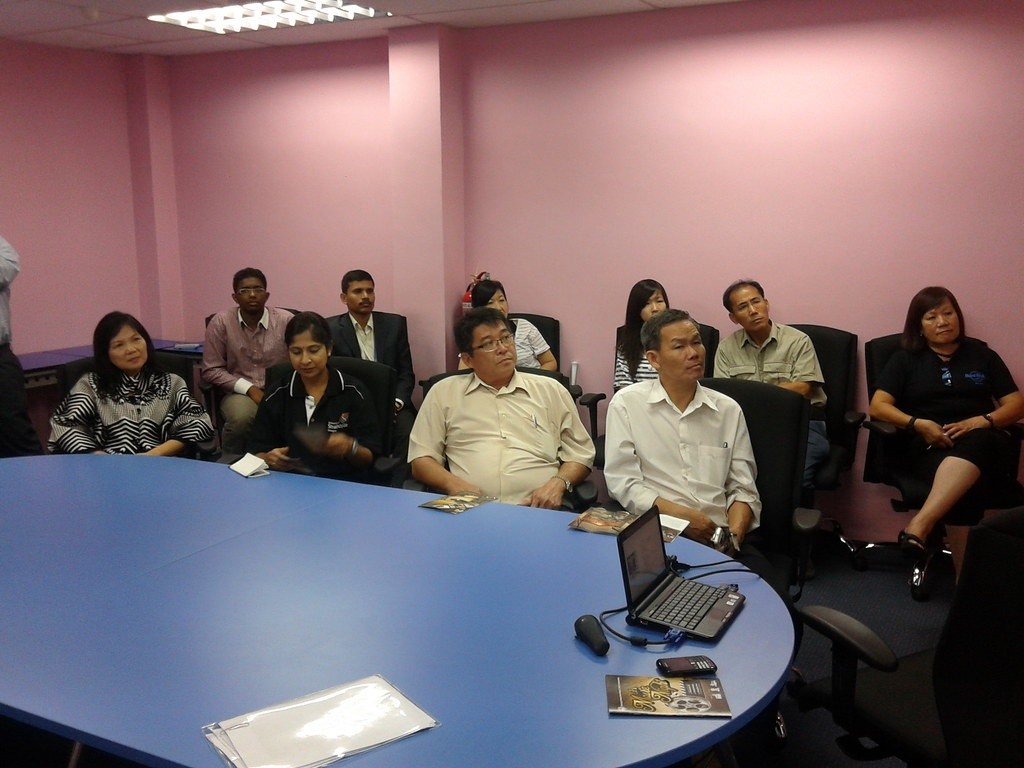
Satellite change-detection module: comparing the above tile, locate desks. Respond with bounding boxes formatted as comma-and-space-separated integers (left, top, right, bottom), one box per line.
16, 337, 204, 390
0, 454, 797, 768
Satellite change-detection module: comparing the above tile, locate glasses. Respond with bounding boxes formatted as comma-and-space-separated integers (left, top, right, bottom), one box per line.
472, 333, 514, 352
236, 288, 265, 295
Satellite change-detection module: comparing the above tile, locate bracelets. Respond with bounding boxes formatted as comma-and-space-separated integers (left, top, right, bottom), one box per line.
906, 416, 917, 434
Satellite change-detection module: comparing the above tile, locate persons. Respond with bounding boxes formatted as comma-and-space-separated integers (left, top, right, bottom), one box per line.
604, 309, 804, 663
713, 280, 830, 579
47, 311, 215, 457
612, 279, 670, 390
202, 268, 296, 454
867, 286, 1024, 585
246, 312, 385, 488
0, 237, 44, 458
324, 269, 418, 489
458, 280, 557, 371
407, 306, 595, 509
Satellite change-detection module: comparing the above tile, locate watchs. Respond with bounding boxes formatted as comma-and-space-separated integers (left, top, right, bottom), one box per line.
982, 413, 994, 428
347, 439, 358, 459
551, 474, 574, 495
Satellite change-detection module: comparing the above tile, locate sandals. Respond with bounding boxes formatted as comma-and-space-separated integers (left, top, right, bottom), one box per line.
897, 529, 926, 556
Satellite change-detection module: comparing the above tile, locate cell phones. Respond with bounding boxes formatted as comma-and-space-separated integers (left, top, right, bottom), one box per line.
656, 655, 717, 677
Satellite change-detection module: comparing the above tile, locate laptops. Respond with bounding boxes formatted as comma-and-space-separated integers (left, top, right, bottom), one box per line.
616, 505, 747, 643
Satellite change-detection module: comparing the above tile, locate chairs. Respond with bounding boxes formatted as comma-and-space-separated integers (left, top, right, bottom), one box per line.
195, 308, 1024, 768
